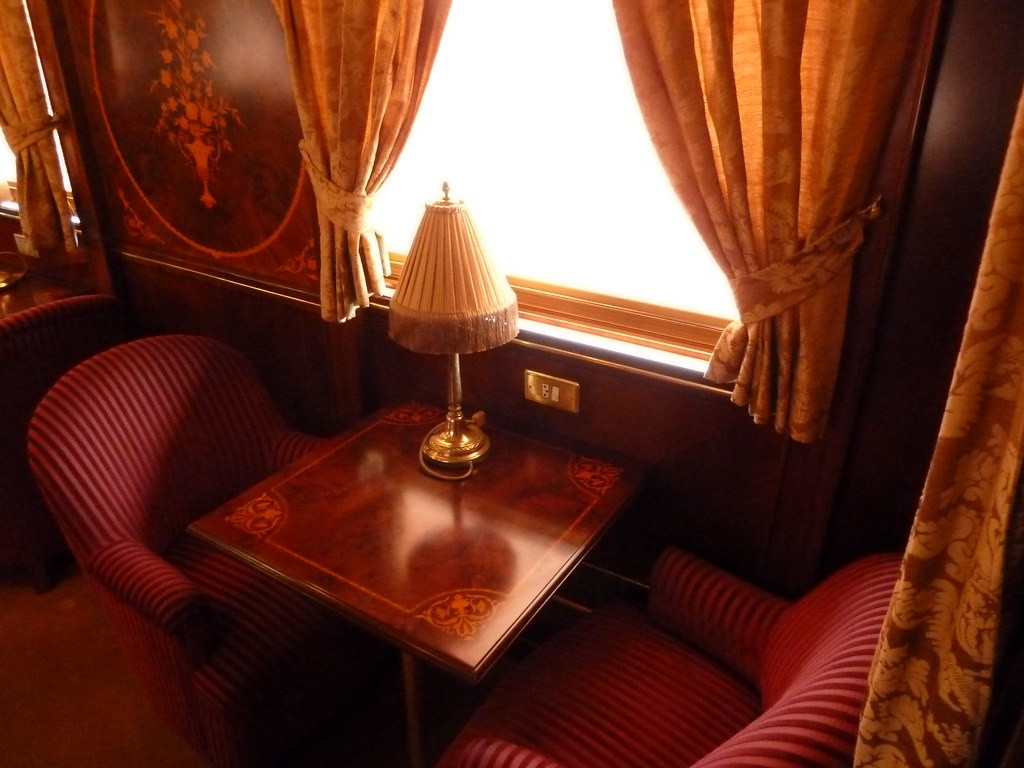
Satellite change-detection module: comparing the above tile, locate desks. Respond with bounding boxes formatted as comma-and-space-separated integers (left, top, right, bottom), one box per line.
184, 395, 654, 768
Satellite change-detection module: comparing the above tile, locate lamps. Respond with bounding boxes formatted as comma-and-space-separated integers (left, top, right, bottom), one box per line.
384, 181, 518, 479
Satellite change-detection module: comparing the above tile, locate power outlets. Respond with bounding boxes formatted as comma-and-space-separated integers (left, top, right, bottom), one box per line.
524, 369, 580, 415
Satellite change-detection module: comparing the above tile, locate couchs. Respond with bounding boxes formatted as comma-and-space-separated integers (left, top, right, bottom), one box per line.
27, 337, 355, 768
0, 293, 126, 593
439, 548, 907, 768
